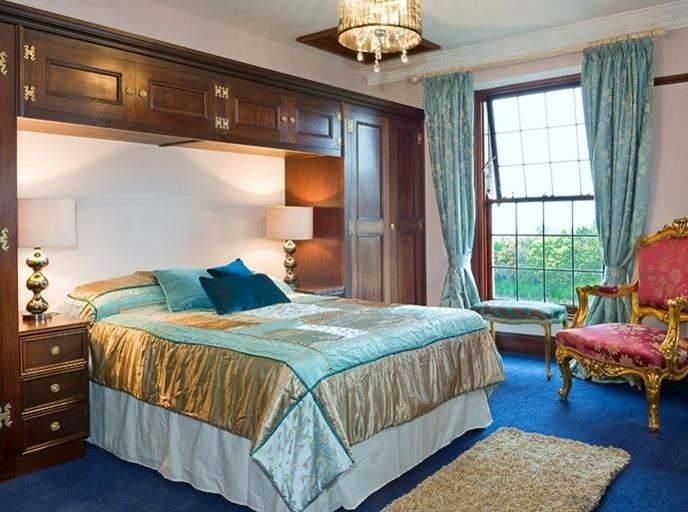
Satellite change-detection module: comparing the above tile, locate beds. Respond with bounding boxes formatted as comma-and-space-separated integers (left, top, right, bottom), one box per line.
55, 269, 506, 512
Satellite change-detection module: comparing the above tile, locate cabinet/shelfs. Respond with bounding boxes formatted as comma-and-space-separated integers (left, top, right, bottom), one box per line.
283, 103, 424, 304
159, 79, 339, 162
18, 28, 221, 149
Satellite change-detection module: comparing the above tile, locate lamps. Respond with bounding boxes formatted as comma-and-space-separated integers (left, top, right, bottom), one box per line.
336, 0, 422, 73
264, 205, 315, 289
16, 198, 75, 322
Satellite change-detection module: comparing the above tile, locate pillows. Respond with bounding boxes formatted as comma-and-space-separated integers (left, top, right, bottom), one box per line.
154, 266, 212, 313
208, 259, 253, 278
199, 272, 289, 314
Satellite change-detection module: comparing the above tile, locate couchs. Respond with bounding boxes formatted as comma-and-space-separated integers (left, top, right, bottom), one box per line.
554, 216, 688, 435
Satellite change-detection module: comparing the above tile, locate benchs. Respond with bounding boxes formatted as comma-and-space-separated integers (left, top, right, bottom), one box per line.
466, 301, 567, 379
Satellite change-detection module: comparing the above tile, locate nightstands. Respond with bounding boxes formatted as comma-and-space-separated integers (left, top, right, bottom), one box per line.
19, 312, 91, 477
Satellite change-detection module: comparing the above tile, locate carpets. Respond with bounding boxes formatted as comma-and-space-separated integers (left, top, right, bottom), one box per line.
375, 423, 631, 512
